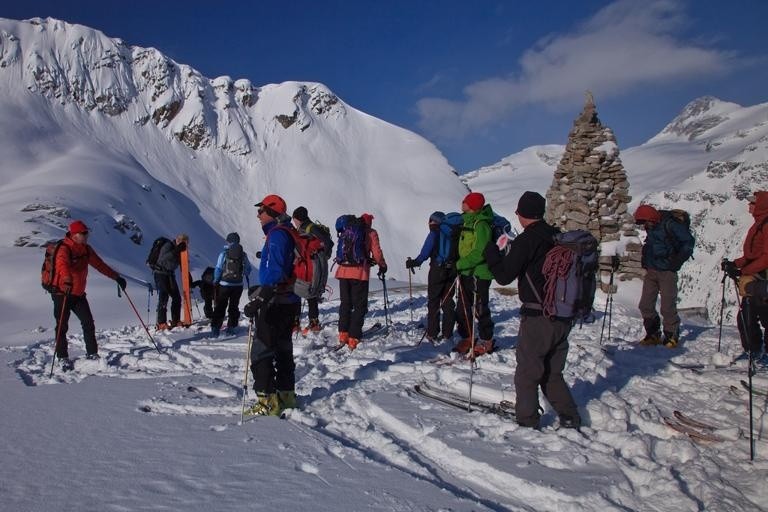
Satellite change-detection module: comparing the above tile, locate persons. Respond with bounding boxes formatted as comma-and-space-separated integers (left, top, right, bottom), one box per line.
290, 206, 331, 332
152, 233, 191, 329
406, 193, 496, 359
632, 202, 696, 351
480, 191, 582, 429
199, 233, 252, 335
239, 195, 305, 419
736, 273, 768, 302
730, 188, 768, 370
48, 219, 128, 373
335, 212, 388, 351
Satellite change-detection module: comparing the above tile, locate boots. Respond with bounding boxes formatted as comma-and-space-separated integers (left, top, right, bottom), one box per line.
277, 390, 297, 409
245, 391, 280, 415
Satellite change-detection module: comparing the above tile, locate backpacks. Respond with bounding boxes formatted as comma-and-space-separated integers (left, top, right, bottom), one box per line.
331, 215, 370, 272
221, 242, 244, 283
473, 215, 518, 257
662, 209, 695, 261
436, 212, 465, 265
305, 220, 334, 260
146, 237, 175, 274
266, 225, 328, 299
526, 228, 599, 323
42, 239, 73, 291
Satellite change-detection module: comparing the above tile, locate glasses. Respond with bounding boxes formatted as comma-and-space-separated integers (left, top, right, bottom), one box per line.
258, 209, 266, 215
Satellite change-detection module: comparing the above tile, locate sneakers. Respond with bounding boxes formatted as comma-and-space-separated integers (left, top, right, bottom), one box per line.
639, 329, 662, 345
158, 317, 239, 339
86, 353, 100, 359
292, 317, 358, 349
756, 354, 768, 367
737, 351, 761, 360
58, 357, 73, 370
426, 330, 494, 356
663, 333, 678, 347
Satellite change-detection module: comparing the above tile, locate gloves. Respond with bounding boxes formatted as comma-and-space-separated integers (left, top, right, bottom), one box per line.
448, 261, 460, 273
368, 256, 376, 266
721, 261, 736, 272
379, 265, 387, 273
172, 241, 186, 257
116, 276, 126, 290
64, 281, 73, 295
244, 284, 275, 316
406, 260, 416, 268
213, 280, 219, 286
725, 266, 742, 276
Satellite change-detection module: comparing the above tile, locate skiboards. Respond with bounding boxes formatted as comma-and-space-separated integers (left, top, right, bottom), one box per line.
729, 379, 767, 400
181, 249, 192, 326
665, 411, 730, 444
667, 359, 750, 376
414, 382, 563, 431
332, 323, 391, 353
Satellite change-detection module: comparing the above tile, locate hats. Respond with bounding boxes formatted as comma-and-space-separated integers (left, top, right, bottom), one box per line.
70, 221, 90, 234
745, 195, 757, 203
254, 195, 286, 215
517, 191, 545, 219
227, 232, 240, 243
430, 211, 445, 224
293, 207, 307, 221
465, 193, 484, 210
